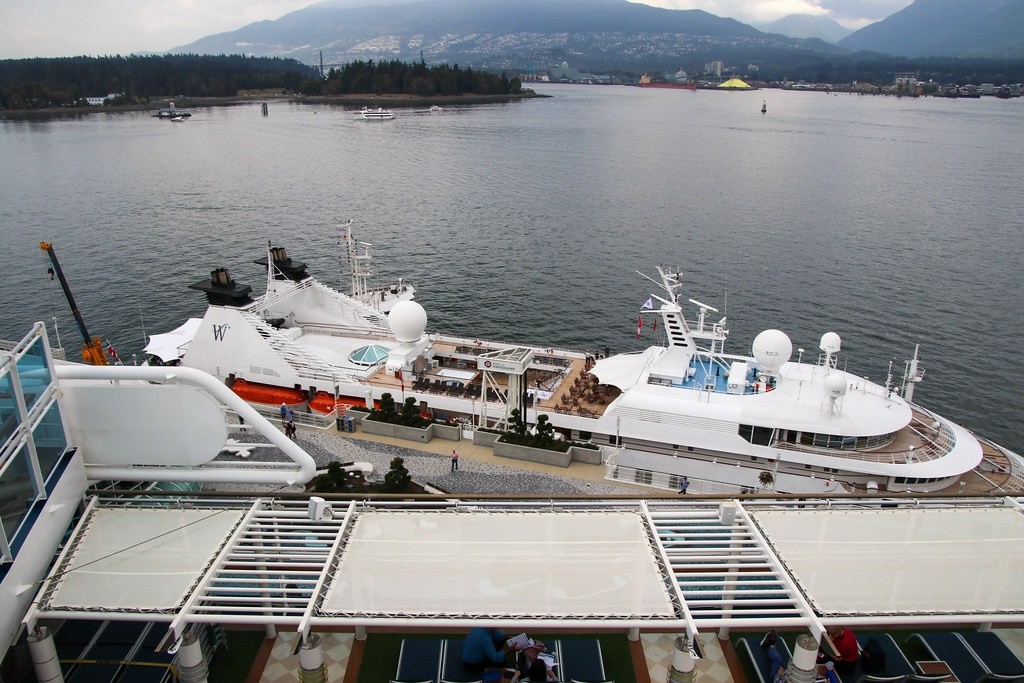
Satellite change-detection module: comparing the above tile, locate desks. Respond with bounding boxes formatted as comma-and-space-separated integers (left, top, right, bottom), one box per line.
915, 661, 961, 683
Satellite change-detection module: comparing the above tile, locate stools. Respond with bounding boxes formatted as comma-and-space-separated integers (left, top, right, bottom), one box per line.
688, 368, 696, 377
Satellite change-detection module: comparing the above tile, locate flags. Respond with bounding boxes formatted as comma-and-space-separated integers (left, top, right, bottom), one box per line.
637, 316, 642, 338
106, 341, 118, 361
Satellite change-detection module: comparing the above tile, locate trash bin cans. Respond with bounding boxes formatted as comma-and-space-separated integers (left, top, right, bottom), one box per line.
336, 415, 356, 433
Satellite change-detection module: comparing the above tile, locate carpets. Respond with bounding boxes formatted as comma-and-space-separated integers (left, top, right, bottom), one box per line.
730, 628, 979, 683
357, 622, 638, 683
207, 630, 268, 683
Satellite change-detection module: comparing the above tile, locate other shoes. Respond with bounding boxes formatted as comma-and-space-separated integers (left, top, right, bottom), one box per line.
451, 470, 453, 471
456, 467, 457, 470
294, 437, 296, 438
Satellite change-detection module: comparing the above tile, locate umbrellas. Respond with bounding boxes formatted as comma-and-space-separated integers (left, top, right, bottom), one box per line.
587, 354, 642, 392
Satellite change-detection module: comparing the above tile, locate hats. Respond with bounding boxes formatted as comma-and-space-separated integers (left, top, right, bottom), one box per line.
282, 403, 286, 406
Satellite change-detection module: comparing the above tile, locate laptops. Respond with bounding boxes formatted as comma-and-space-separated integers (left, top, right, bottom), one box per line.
537, 652, 555, 678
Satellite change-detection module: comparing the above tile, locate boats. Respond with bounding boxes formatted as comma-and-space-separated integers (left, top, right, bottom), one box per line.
137, 220, 1024, 496
760, 108, 767, 113
353, 106, 395, 120
171, 116, 184, 121
430, 106, 441, 111
151, 108, 192, 117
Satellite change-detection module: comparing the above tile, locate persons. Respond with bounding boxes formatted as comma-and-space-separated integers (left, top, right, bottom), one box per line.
462, 628, 557, 683
585, 349, 604, 363
679, 477, 689, 494
449, 451, 458, 471
547, 348, 553, 353
239, 416, 246, 431
762, 625, 859, 683
281, 402, 296, 439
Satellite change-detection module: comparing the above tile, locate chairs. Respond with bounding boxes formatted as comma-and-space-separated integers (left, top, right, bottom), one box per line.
554, 359, 604, 417
412, 377, 535, 406
17, 593, 229, 683
390, 639, 615, 683
736, 632, 1024, 683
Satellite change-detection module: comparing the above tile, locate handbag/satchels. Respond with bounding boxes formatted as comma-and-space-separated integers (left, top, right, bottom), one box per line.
518, 647, 539, 674
290, 409, 293, 415
291, 427, 296, 431
